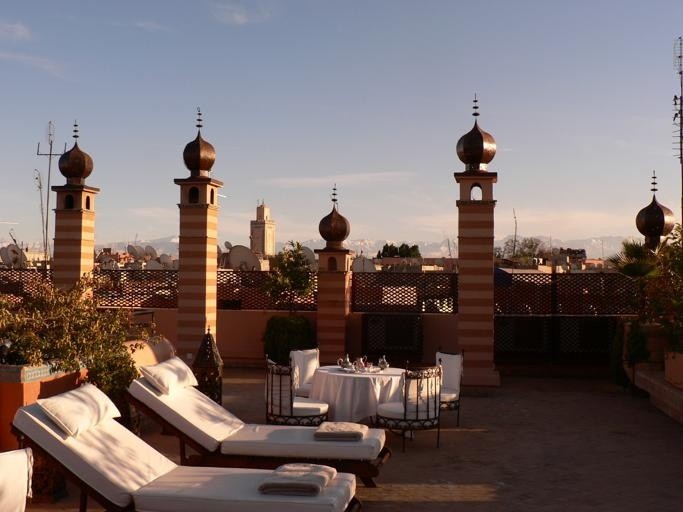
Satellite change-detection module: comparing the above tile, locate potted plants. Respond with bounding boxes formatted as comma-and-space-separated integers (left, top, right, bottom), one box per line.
605, 219, 682, 392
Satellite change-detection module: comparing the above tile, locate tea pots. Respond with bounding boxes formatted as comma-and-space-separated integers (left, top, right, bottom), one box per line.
335, 353, 391, 372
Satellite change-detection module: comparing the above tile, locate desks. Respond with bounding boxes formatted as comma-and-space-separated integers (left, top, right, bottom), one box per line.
312, 365, 415, 427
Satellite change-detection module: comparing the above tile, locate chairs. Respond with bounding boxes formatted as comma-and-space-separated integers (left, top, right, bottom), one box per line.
11, 385, 362, 511
437, 348, 464, 427
127, 356, 391, 488
264, 358, 330, 427
290, 348, 320, 397
376, 366, 444, 454
